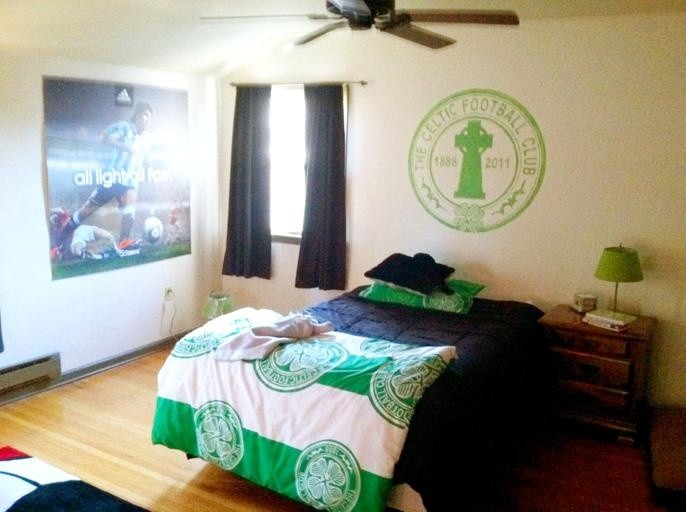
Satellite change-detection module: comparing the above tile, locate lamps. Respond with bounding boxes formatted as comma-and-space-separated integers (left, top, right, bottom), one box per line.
593, 243, 644, 311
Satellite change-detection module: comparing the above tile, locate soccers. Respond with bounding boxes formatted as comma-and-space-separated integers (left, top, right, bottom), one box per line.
143, 217, 162, 244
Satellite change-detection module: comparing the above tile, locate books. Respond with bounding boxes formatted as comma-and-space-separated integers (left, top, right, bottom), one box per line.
585, 307, 639, 327
582, 317, 633, 333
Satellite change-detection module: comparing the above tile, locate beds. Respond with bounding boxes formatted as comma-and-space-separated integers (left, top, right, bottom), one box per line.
154, 284, 546, 512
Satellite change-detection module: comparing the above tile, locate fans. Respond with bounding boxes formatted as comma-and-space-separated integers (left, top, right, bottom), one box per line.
200, 1, 520, 51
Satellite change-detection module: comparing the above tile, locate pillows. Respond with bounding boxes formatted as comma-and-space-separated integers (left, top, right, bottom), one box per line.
359, 252, 486, 315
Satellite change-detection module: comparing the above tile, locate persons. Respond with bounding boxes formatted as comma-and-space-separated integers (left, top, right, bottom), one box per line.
50, 206, 128, 264
56, 101, 153, 258
161, 175, 191, 242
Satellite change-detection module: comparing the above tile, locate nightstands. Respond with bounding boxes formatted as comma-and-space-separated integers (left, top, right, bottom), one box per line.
539, 302, 657, 445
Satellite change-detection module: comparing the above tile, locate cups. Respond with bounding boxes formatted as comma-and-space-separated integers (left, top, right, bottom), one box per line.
572, 292, 598, 312
